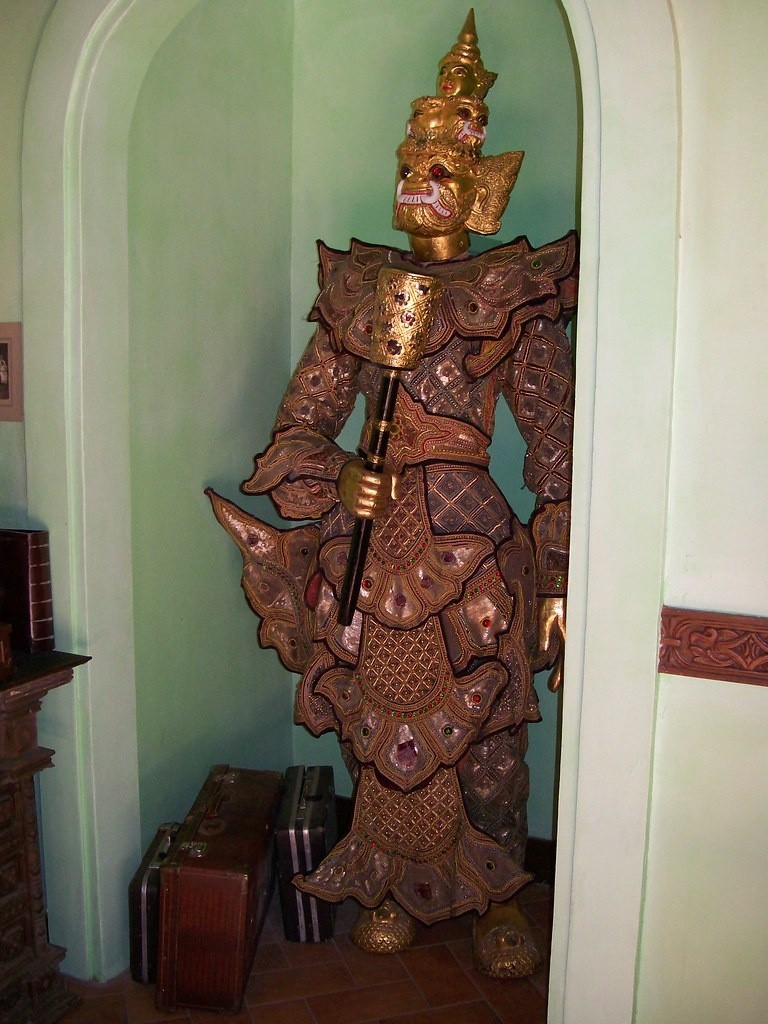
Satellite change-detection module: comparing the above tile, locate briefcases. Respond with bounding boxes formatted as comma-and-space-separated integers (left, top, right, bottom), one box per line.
273, 764, 340, 943
128, 821, 182, 984
158, 765, 285, 1012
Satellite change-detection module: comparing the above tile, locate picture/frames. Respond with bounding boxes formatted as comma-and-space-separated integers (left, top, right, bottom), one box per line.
0, 321, 22, 420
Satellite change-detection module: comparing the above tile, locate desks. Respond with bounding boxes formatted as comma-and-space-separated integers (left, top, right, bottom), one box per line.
0, 651, 87, 1018
